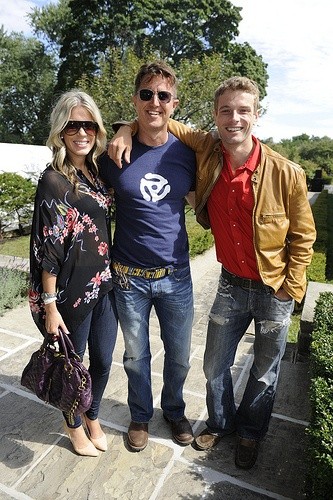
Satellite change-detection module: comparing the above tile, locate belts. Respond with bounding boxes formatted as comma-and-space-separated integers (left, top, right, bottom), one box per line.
221, 268, 273, 291
111, 261, 190, 279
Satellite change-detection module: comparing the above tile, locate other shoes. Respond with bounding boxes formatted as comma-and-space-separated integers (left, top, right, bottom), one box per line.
194, 427, 232, 450
234, 435, 259, 469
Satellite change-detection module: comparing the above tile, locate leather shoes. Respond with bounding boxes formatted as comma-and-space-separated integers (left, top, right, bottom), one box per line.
164, 414, 194, 445
127, 420, 148, 450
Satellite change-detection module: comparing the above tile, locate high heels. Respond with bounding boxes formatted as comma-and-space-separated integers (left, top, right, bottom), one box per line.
66, 426, 99, 456
83, 414, 108, 451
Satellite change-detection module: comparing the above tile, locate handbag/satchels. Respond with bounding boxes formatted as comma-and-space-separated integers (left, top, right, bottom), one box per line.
20, 331, 93, 417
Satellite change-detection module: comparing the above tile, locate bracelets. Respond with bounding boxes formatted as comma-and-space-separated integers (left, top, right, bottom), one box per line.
44, 298, 57, 304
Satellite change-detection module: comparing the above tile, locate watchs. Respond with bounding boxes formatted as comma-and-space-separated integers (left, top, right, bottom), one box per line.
41, 292, 57, 299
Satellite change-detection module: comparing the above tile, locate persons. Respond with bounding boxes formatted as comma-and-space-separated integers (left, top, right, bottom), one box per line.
29, 90, 119, 456
94, 62, 198, 451
107, 75, 316, 469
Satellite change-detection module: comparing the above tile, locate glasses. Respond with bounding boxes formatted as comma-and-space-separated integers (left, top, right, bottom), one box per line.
135, 88, 175, 104
62, 120, 99, 137
